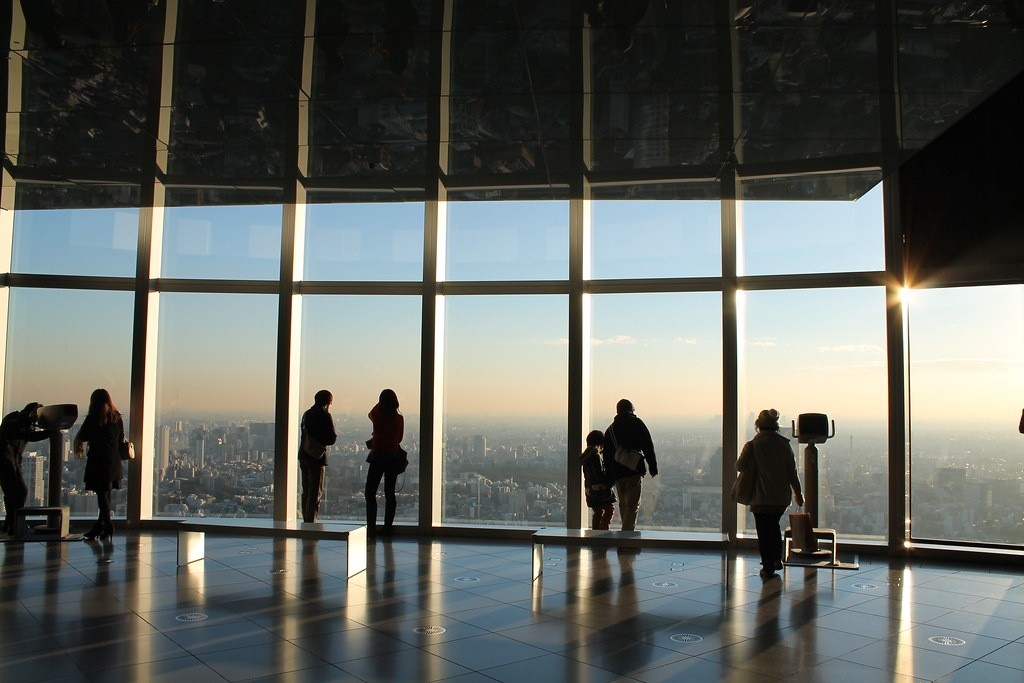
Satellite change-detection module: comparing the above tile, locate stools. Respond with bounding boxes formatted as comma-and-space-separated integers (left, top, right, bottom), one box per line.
784, 528, 840, 566
25, 506, 69, 537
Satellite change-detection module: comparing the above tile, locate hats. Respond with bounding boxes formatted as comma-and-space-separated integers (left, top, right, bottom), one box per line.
755, 408, 780, 430
617, 399, 635, 415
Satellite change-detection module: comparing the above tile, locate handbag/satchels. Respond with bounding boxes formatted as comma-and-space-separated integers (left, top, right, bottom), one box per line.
609, 424, 642, 471
119, 434, 136, 461
375, 447, 408, 474
301, 412, 326, 461
731, 441, 787, 508
789, 504, 819, 549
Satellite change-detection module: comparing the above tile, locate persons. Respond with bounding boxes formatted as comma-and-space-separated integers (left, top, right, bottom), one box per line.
298, 390, 337, 523
734, 409, 805, 576
73, 389, 125, 540
0, 402, 50, 536
578, 399, 658, 531
364, 389, 404, 525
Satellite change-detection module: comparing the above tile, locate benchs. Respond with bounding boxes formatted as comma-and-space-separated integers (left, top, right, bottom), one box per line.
532, 525, 731, 587
176, 517, 368, 578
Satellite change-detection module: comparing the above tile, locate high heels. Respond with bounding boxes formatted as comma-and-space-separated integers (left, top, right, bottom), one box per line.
83, 522, 114, 540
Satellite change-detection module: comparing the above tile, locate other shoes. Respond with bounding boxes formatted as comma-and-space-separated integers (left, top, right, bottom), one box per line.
617, 547, 640, 550
759, 561, 784, 580
369, 527, 392, 537
